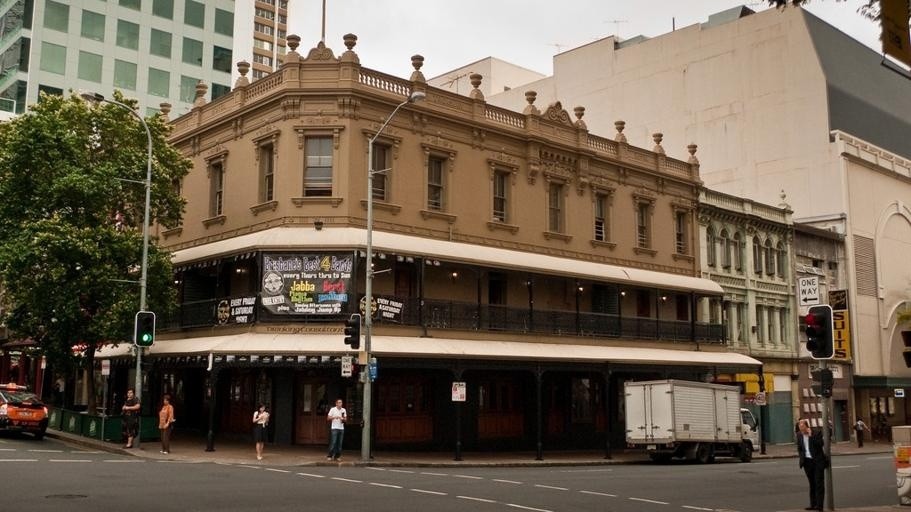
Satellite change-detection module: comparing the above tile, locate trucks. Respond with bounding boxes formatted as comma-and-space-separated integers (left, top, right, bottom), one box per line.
622, 378, 761, 462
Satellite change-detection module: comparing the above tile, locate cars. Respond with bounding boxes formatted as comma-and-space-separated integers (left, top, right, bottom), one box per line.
1, 382, 50, 441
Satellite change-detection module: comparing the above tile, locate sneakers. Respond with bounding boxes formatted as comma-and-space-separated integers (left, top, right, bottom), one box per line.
336, 458, 344, 462
257, 456, 263, 460
328, 457, 332, 461
160, 451, 168, 455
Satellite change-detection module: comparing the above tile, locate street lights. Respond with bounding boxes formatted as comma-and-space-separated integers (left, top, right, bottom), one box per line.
78, 89, 153, 448
356, 89, 428, 463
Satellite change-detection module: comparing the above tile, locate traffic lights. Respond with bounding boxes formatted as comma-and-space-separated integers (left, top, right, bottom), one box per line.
805, 303, 837, 359
344, 313, 362, 349
810, 368, 832, 399
133, 310, 157, 347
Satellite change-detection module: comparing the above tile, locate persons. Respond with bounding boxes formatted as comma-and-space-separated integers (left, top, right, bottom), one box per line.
122, 388, 141, 449
852, 416, 870, 447
252, 402, 270, 460
327, 399, 347, 462
827, 416, 833, 440
797, 419, 826, 511
6, 372, 64, 409
158, 395, 176, 453
795, 417, 804, 435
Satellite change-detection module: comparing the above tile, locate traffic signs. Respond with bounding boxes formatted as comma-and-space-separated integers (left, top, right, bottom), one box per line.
341, 356, 354, 378
799, 275, 819, 306
